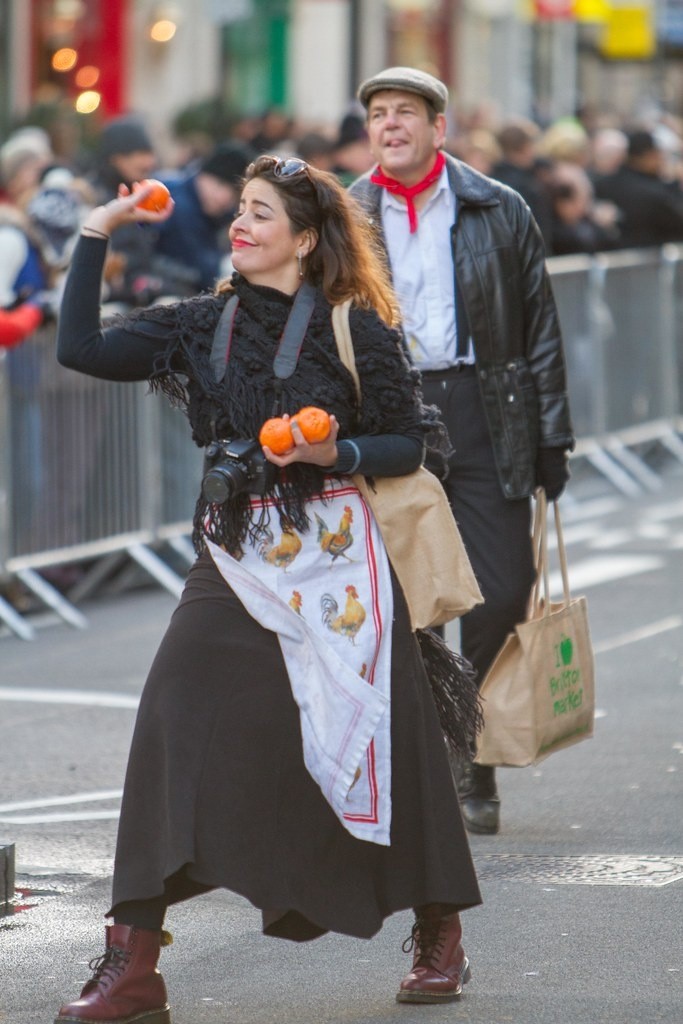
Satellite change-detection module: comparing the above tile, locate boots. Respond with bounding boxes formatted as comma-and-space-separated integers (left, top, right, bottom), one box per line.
398, 904, 471, 1003
55, 924, 174, 1024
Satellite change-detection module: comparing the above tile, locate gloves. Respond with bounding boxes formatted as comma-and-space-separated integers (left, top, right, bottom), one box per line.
532, 446, 570, 501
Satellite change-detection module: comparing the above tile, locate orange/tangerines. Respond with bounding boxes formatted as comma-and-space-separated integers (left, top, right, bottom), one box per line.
136, 179, 170, 211
260, 418, 293, 454
290, 405, 330, 442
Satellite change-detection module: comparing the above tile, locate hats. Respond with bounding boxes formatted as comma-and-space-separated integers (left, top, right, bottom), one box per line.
358, 67, 448, 114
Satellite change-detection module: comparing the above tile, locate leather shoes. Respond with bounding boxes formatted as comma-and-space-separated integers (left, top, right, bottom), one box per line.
447, 742, 500, 834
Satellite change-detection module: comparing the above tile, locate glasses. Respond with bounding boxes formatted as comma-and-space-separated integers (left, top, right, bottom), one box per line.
274, 155, 313, 183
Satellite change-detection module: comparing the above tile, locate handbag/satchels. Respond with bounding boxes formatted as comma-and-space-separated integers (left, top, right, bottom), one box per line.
332, 298, 485, 632
473, 487, 594, 766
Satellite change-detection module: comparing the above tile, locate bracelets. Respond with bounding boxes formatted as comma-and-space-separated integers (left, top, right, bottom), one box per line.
81, 223, 112, 243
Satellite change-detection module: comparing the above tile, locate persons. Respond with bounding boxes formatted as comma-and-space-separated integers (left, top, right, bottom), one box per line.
52, 151, 482, 1023
328, 62, 574, 836
0, 81, 683, 573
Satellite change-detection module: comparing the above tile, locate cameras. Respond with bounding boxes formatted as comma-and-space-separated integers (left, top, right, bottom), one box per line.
201, 437, 278, 504
